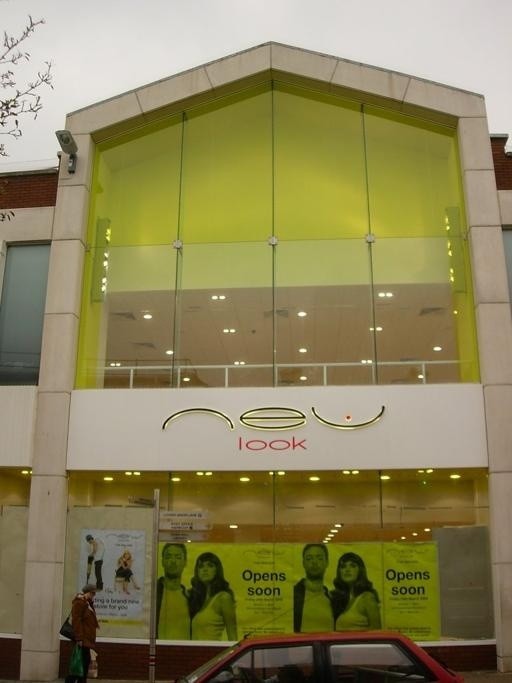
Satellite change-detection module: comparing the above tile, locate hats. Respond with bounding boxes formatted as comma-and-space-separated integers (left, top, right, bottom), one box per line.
82, 584, 96, 593
86, 535, 92, 542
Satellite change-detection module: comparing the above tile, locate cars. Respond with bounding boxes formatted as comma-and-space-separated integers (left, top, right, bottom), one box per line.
174, 632, 464, 683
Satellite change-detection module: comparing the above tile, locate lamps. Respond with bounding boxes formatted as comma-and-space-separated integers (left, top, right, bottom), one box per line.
52, 128, 82, 174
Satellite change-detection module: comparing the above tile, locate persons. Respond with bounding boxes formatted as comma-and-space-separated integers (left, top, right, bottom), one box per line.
231, 661, 308, 683
114, 549, 141, 595
186, 551, 238, 641
293, 544, 334, 634
157, 543, 192, 639
85, 534, 105, 591
328, 552, 381, 630
65, 584, 100, 683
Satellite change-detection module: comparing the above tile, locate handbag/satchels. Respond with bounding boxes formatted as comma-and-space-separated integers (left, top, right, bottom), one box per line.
69, 645, 84, 677
59, 621, 73, 640
